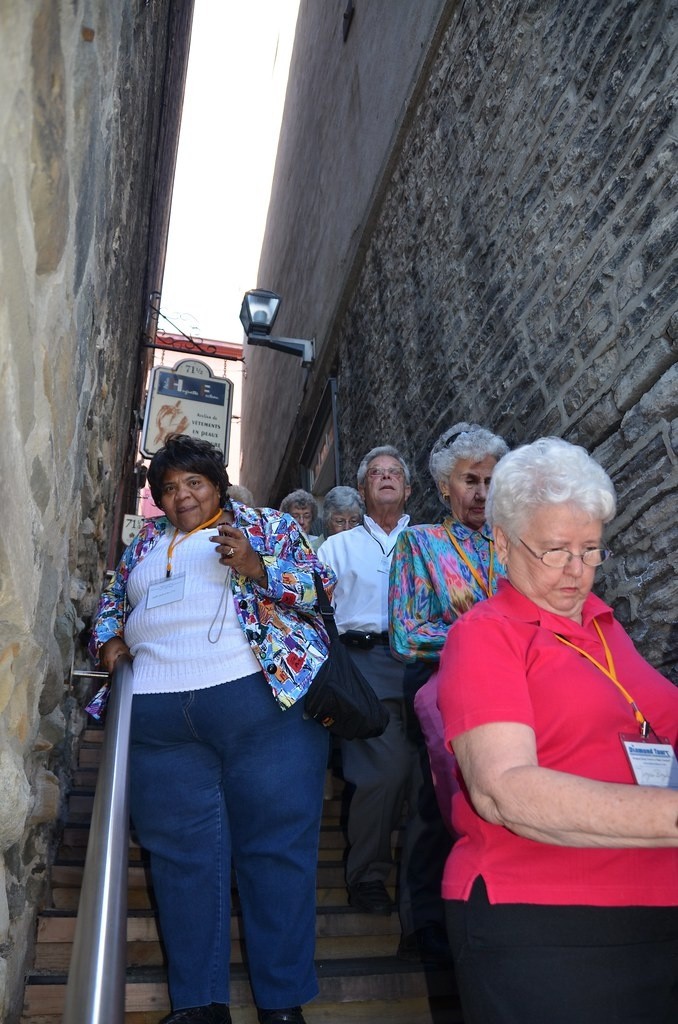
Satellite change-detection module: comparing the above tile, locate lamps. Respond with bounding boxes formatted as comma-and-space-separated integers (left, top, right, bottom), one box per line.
238, 287, 316, 371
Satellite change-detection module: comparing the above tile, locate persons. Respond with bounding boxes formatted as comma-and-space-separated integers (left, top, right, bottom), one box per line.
280, 490, 325, 552
85, 431, 339, 1024
225, 484, 255, 506
309, 486, 365, 554
316, 445, 412, 916
387, 421, 512, 969
416, 431, 678, 1024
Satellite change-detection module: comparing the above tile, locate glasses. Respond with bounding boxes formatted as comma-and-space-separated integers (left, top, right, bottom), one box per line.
366, 466, 407, 477
443, 431, 469, 447
331, 517, 362, 527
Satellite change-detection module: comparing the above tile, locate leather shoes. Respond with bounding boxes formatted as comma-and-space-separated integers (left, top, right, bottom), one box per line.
258, 1007, 306, 1024
349, 880, 394, 912
398, 932, 447, 965
161, 1004, 232, 1024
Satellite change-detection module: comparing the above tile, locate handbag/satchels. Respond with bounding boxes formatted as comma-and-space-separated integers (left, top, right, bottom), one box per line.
294, 569, 392, 744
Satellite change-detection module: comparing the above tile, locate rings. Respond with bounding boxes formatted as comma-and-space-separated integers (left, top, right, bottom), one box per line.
226, 547, 234, 558
